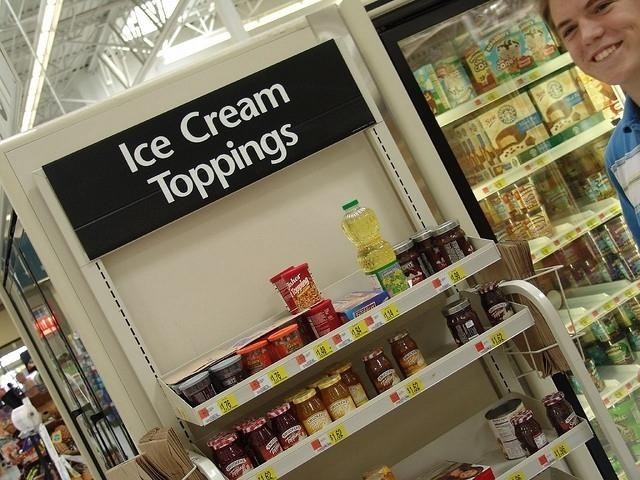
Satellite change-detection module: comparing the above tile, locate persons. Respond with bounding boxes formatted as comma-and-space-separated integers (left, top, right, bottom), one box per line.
538, 0, 640, 252
0, 349, 46, 409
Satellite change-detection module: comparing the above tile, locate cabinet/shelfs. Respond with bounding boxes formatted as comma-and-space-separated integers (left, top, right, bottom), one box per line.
158, 227, 640, 480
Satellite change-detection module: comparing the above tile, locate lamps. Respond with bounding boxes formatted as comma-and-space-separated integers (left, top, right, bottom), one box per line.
1, 1, 72, 244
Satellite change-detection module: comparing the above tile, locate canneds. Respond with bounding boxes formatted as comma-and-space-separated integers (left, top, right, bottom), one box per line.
207, 402, 308, 480
393, 218, 476, 288
389, 329, 427, 378
283, 360, 369, 436
510, 411, 547, 455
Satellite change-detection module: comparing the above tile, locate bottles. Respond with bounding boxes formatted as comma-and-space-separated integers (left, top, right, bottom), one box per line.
203, 361, 370, 480
507, 408, 549, 455
393, 219, 476, 287
340, 197, 410, 302
385, 328, 429, 379
